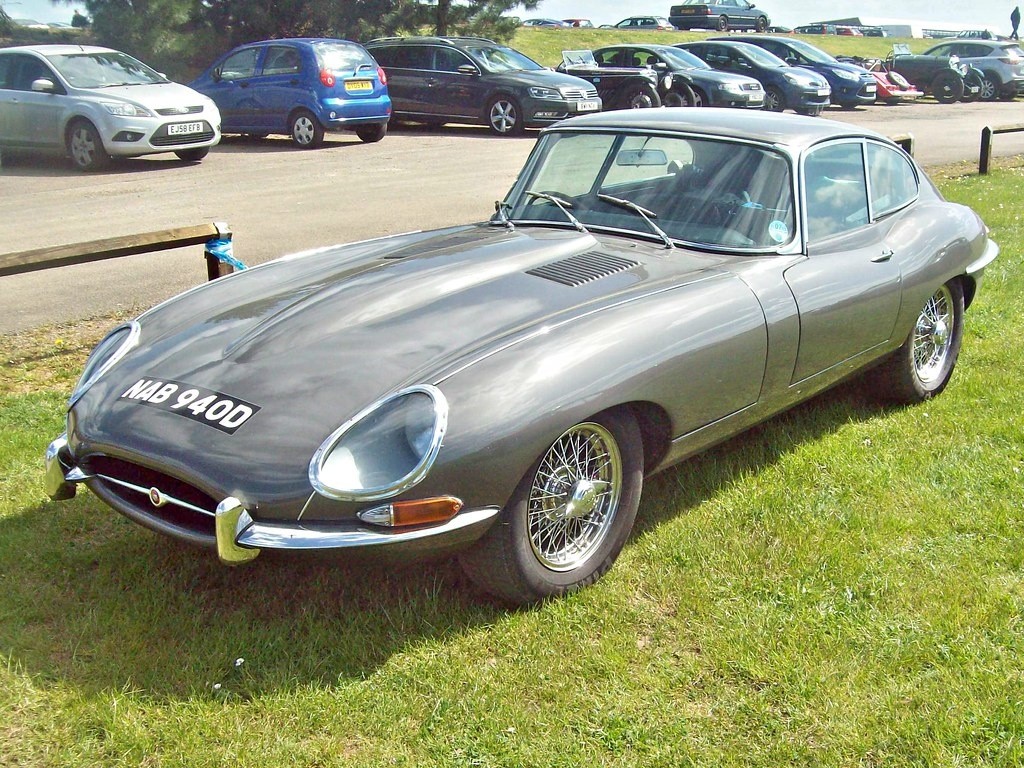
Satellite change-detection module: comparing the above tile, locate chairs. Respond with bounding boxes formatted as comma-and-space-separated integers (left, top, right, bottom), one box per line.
633, 57, 641, 67
647, 56, 657, 64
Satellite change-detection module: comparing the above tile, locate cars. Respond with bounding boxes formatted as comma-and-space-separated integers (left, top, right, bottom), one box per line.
187, 38, 392, 150
521, 0, 1011, 43
862, 41, 985, 103
556, 50, 696, 107
0, 43, 221, 171
835, 43, 924, 106
43, 105, 995, 608
363, 35, 602, 136
673, 41, 832, 117
578, 44, 766, 109
706, 35, 877, 110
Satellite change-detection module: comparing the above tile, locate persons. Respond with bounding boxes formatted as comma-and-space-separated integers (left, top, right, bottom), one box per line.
1010, 6, 1020, 40
71, 9, 91, 27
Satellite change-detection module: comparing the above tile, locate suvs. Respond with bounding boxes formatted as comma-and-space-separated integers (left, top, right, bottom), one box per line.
919, 40, 1024, 102
